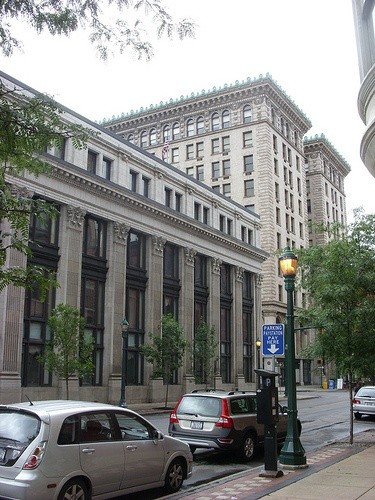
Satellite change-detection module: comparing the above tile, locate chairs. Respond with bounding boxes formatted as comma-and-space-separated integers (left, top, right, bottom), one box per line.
80, 419, 108, 441
232, 402, 248, 413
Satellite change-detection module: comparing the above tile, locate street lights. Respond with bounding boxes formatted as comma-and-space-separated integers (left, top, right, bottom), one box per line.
119, 317, 130, 407
256, 337, 262, 389
279, 246, 307, 466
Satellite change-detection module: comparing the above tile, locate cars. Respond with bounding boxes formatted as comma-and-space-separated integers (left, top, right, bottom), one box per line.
353, 386, 375, 419
0, 399, 194, 500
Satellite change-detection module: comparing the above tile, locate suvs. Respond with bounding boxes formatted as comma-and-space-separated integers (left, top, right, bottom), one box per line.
168, 388, 301, 461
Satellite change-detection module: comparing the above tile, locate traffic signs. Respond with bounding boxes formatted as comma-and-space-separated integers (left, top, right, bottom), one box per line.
262, 325, 285, 356
